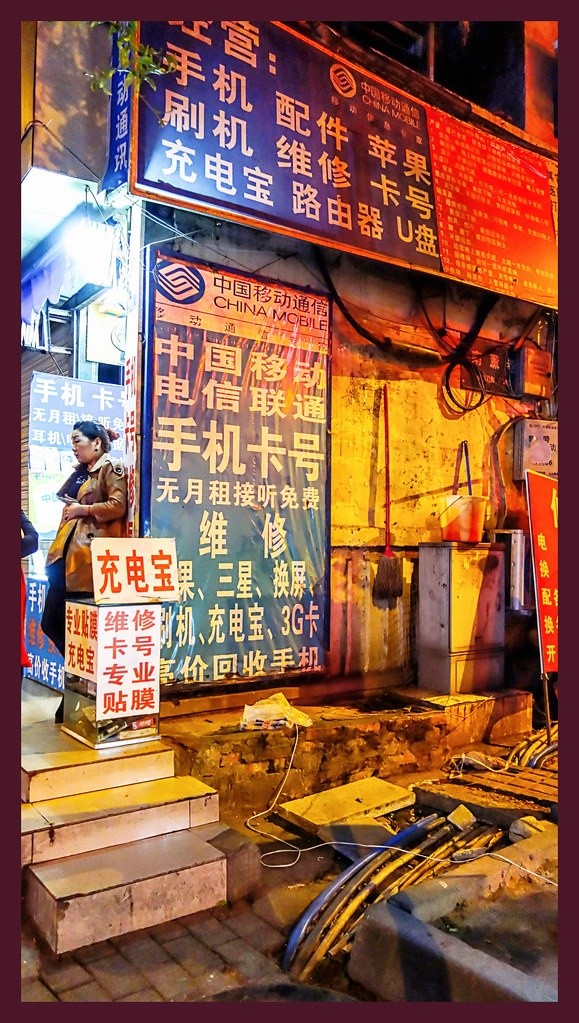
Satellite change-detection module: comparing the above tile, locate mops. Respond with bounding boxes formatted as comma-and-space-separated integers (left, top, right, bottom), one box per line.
372, 384, 403, 602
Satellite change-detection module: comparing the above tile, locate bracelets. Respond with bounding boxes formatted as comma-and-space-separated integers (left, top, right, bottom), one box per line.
88, 505, 91, 516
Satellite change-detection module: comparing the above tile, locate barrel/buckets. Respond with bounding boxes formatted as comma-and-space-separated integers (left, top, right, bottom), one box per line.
437, 493, 489, 543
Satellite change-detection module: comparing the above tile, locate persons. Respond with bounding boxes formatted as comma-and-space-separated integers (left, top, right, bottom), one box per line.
21, 507, 39, 698
40, 419, 128, 725
